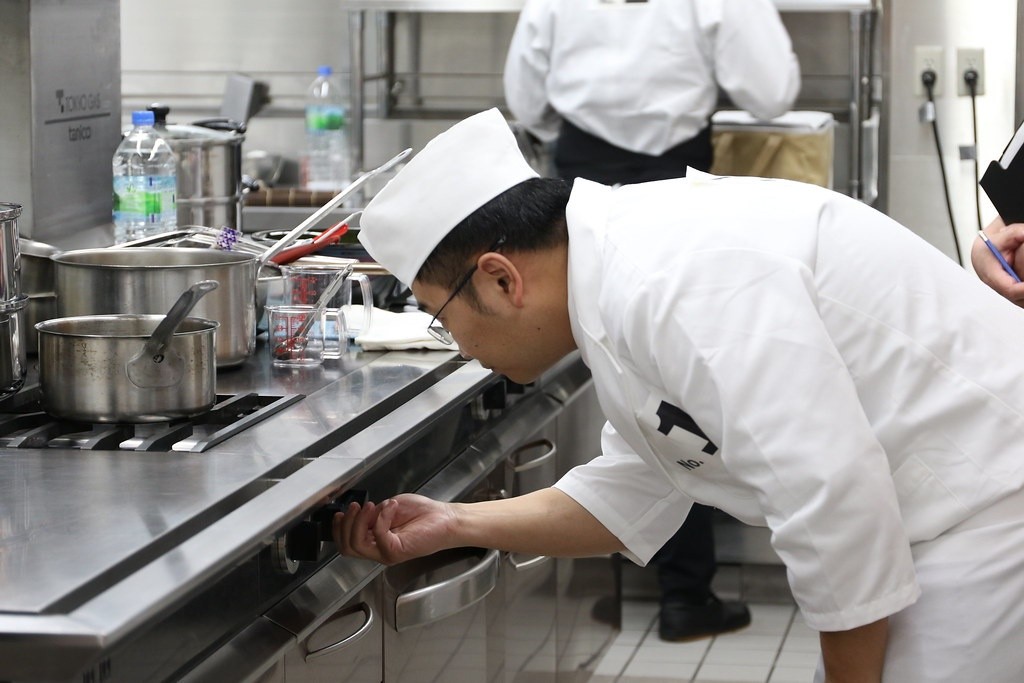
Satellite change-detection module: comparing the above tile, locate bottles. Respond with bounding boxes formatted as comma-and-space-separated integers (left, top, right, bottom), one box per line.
305, 66, 347, 190
112, 110, 177, 244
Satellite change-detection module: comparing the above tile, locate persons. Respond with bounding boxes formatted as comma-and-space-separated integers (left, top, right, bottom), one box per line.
503, 0, 804, 643
331, 107, 1024, 682
970, 118, 1023, 304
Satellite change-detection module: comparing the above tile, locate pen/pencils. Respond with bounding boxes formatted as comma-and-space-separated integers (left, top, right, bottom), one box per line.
977, 228, 1022, 284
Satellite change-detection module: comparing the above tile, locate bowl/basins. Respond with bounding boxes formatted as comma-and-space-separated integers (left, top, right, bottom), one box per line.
20, 238, 62, 296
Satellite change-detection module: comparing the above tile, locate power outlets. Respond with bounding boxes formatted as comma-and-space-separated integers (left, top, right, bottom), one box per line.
957, 48, 984, 95
913, 46, 945, 96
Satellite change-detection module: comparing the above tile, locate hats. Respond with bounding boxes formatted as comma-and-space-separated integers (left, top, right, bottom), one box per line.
355, 108, 541, 290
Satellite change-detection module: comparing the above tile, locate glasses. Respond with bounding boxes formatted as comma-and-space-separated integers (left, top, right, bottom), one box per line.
427, 233, 511, 346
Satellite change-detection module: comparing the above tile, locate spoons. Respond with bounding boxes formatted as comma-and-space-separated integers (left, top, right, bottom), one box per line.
273, 264, 353, 360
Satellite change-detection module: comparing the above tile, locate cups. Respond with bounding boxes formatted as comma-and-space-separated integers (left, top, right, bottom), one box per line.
277, 263, 373, 359
264, 305, 347, 368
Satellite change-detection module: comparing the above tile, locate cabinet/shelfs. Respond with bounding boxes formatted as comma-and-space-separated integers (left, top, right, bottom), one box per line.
342, 0, 890, 607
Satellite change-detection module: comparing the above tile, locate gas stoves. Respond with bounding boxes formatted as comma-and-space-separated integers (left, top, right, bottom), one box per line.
0, 381, 306, 454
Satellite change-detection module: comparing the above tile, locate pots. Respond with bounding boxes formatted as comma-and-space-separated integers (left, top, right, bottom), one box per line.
120, 104, 260, 234
49, 148, 413, 367
34, 280, 221, 423
0, 201, 30, 402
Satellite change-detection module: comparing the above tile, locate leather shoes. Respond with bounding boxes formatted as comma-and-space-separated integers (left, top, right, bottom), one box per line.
659, 599, 751, 642
592, 596, 621, 631
